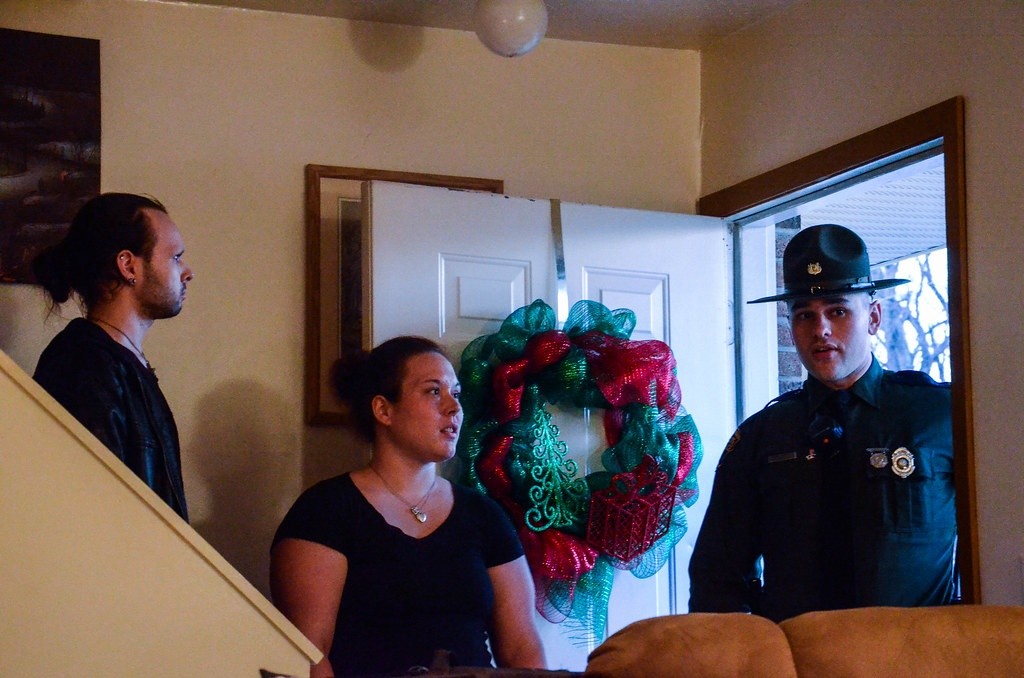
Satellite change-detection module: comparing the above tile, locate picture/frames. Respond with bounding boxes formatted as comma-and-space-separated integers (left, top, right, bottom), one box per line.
304, 163, 506, 431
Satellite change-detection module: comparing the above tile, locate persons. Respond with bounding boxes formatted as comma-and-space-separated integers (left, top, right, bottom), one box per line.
268, 335, 546, 678
32, 192, 189, 521
688, 225, 960, 623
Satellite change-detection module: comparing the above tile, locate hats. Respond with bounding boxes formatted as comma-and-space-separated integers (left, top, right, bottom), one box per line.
746, 224, 911, 305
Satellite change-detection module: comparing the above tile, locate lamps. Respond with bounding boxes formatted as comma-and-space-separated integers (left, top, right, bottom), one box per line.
474, 0, 549, 59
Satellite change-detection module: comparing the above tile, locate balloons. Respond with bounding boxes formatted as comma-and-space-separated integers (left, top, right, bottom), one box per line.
473, 0, 549, 58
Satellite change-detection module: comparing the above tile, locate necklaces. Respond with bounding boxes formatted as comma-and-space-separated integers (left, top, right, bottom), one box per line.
92, 316, 150, 368
371, 463, 436, 523
